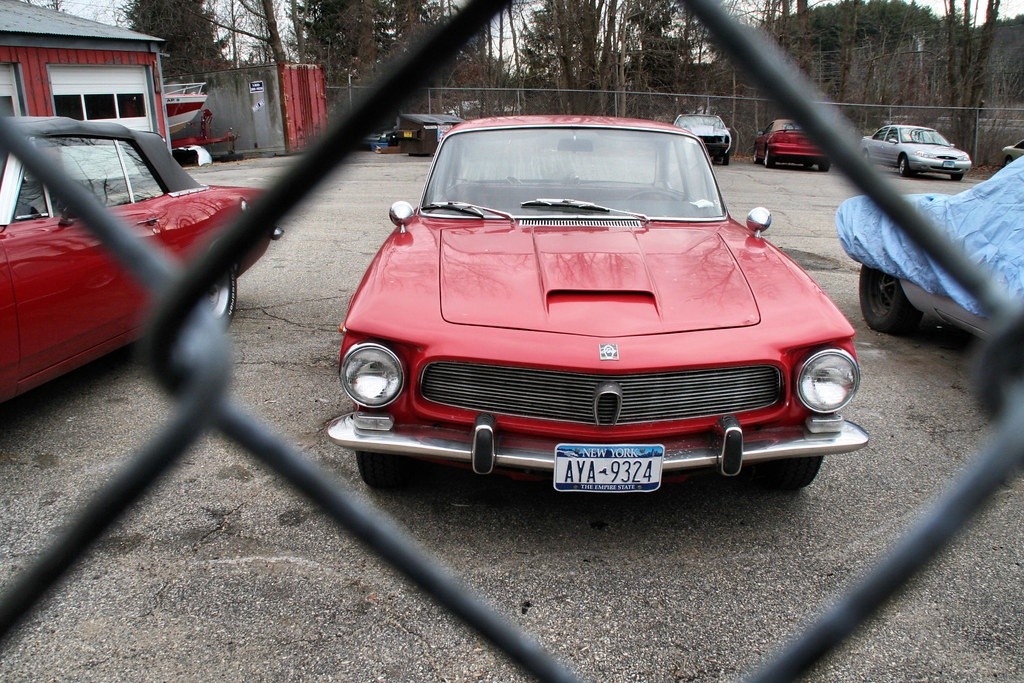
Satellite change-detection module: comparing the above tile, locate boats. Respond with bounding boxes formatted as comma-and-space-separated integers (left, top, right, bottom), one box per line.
163, 80, 209, 133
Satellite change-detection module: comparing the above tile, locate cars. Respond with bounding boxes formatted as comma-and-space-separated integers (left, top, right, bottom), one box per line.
859, 124, 973, 182
671, 112, 734, 167
1000, 138, 1024, 167
0, 111, 287, 411
832, 158, 1023, 348
325, 114, 872, 498
752, 118, 833, 173
